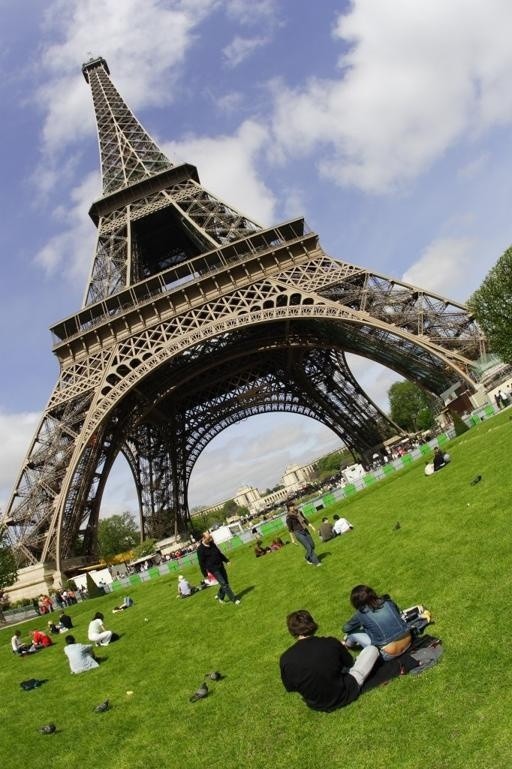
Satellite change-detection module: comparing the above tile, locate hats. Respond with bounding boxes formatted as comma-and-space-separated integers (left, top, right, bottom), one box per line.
178, 574, 186, 581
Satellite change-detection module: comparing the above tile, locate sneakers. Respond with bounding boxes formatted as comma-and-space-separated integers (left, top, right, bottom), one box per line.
234, 599, 241, 605
214, 595, 225, 604
304, 555, 313, 566
315, 562, 323, 567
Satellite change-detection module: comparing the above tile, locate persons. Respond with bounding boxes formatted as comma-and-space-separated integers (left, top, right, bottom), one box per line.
363, 382, 512, 476
1, 530, 220, 656
65, 636, 101, 676
197, 532, 241, 606
286, 502, 325, 568
241, 478, 354, 558
278, 610, 380, 713
343, 584, 413, 662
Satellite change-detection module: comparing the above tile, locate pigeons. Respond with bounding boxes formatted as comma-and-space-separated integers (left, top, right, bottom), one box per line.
210, 671, 221, 680
144, 616, 150, 623
189, 682, 208, 703
392, 521, 401, 531
94, 698, 110, 713
35, 722, 56, 735
